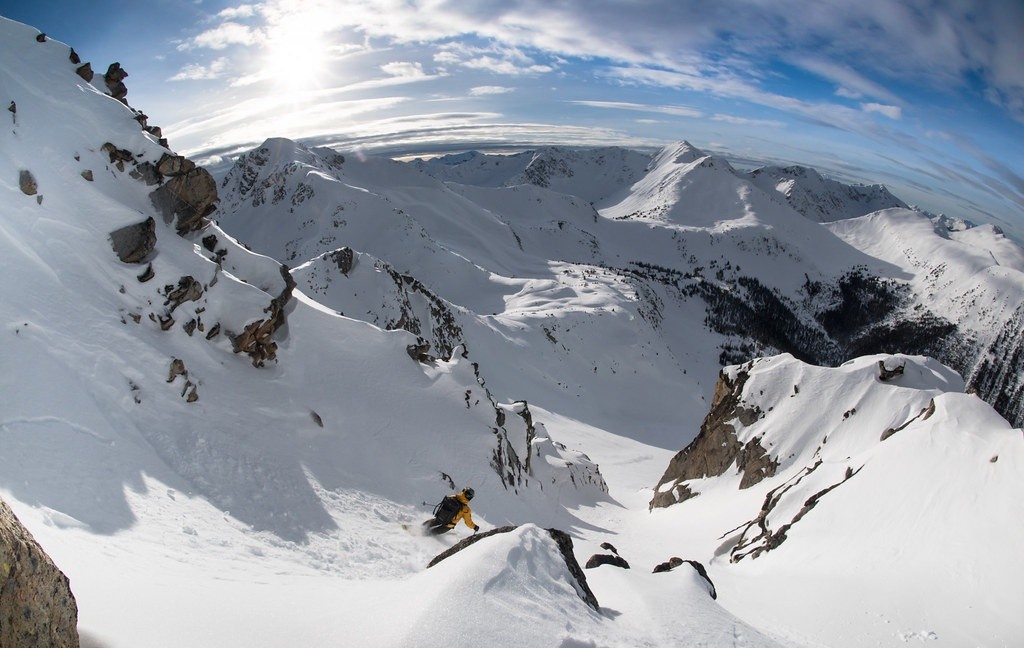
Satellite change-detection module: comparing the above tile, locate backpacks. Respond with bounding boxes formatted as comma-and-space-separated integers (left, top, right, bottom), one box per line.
433, 495, 467, 527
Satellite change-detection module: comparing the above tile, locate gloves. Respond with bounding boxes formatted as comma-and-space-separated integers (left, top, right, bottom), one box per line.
474, 525, 479, 531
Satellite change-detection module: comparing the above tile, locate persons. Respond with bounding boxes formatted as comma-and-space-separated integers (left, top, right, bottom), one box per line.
423, 487, 479, 536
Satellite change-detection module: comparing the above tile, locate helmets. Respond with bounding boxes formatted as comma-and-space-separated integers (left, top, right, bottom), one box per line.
462, 487, 475, 500
